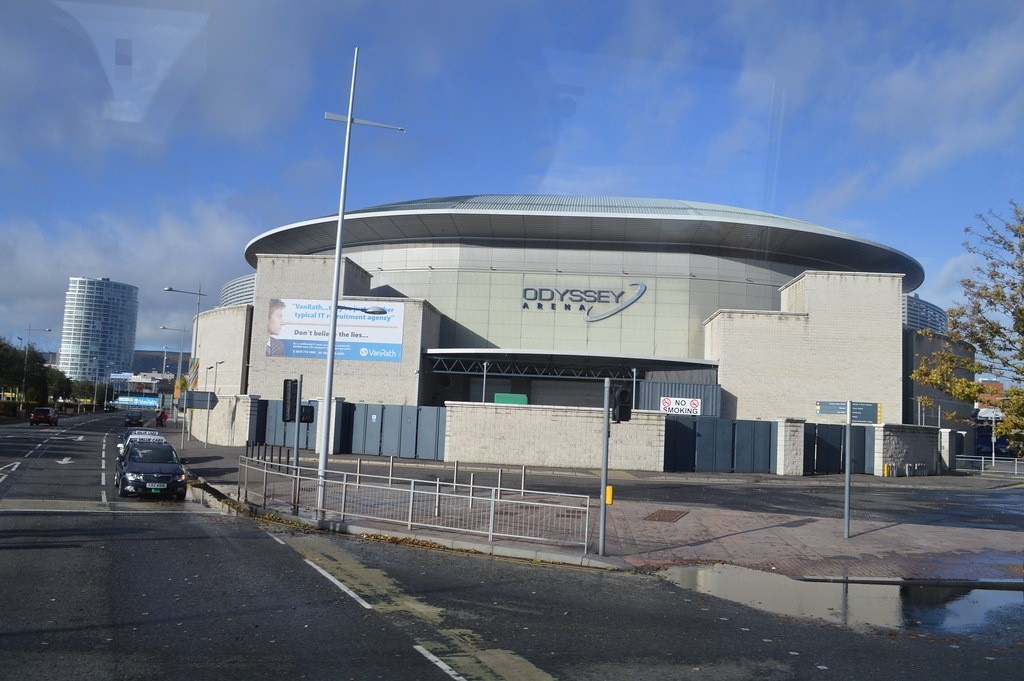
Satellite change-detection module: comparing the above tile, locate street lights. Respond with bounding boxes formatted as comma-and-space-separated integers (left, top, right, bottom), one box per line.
19, 323, 51, 410
92, 366, 110, 413
164, 283, 208, 441
163, 346, 168, 378
160, 325, 189, 428
104, 371, 118, 412
316, 46, 406, 520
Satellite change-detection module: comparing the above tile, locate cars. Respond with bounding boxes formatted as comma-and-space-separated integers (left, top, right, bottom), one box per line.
116, 427, 166, 458
122, 409, 146, 427
29, 407, 59, 427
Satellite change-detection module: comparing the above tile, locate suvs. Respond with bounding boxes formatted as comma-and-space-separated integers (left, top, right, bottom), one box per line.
114, 441, 189, 501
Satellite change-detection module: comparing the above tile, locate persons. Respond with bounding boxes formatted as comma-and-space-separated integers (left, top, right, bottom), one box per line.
155, 410, 168, 428
267, 298, 286, 356
